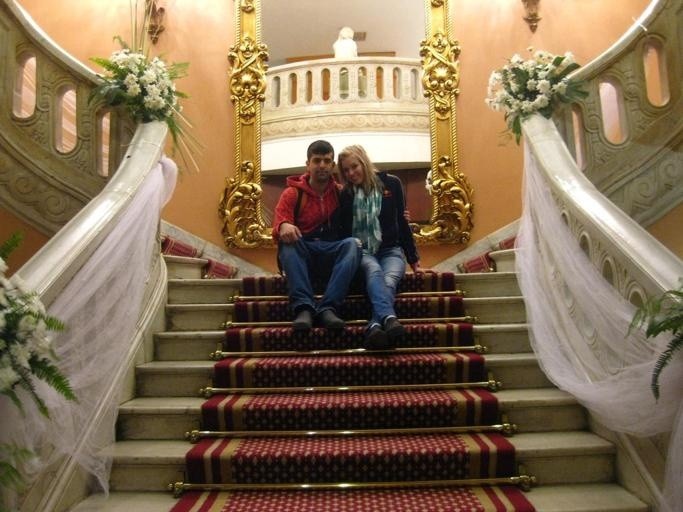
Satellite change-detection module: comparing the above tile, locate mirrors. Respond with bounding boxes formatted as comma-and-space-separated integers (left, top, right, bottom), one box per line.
211, 0, 468, 247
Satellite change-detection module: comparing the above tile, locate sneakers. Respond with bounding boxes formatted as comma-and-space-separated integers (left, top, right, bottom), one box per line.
363, 325, 388, 349
318, 309, 345, 329
385, 315, 404, 338
293, 310, 312, 330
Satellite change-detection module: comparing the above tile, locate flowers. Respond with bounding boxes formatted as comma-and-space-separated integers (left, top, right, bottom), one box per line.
0, 231, 79, 423
482, 44, 583, 149
87, 2, 197, 177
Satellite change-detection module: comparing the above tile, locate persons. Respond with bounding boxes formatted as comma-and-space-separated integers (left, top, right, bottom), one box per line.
329, 144, 421, 350
269, 139, 412, 333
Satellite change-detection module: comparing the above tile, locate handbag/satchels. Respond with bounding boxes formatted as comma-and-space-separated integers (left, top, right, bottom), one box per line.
370, 217, 400, 244
